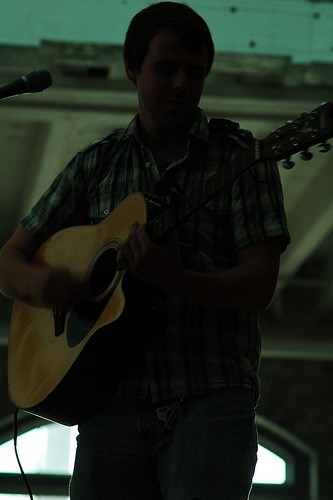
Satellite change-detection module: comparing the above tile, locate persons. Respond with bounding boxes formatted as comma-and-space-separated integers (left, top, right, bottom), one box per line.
0, 3, 291, 500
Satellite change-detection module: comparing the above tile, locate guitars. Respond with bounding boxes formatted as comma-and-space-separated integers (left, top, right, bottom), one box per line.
8, 101, 333, 426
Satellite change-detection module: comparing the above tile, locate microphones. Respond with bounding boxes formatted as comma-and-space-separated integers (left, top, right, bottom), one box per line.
0, 68, 52, 101
